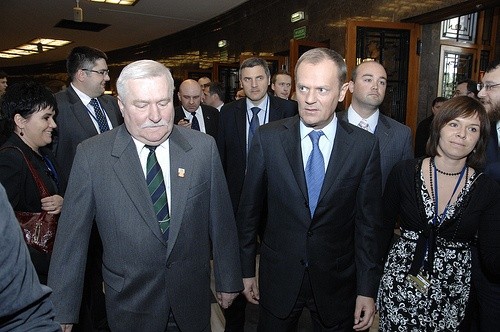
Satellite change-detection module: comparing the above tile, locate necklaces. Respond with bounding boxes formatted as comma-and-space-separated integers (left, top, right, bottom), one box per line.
429, 153, 469, 247
85, 97, 111, 132
245, 95, 269, 127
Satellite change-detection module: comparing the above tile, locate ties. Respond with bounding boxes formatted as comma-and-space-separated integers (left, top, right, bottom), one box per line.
305, 129, 328, 218
89, 98, 110, 134
359, 120, 370, 132
191, 112, 200, 132
144, 143, 170, 241
247, 107, 262, 159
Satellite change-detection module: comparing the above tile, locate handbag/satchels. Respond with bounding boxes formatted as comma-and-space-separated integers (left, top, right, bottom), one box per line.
0, 145, 58, 255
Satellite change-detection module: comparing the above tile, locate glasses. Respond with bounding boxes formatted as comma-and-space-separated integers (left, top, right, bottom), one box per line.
476, 82, 500, 90
81, 67, 109, 76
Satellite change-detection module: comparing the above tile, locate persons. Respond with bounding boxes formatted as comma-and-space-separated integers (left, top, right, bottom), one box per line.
198, 76, 246, 115
333, 59, 416, 286
46, 59, 245, 332
170, 78, 220, 142
270, 70, 293, 101
44, 44, 127, 214
476, 58, 500, 332
373, 95, 493, 332
236, 47, 388, 332
214, 56, 303, 332
0, 88, 65, 287
0, 182, 65, 332
413, 94, 449, 158
454, 79, 481, 102
0, 73, 17, 143
